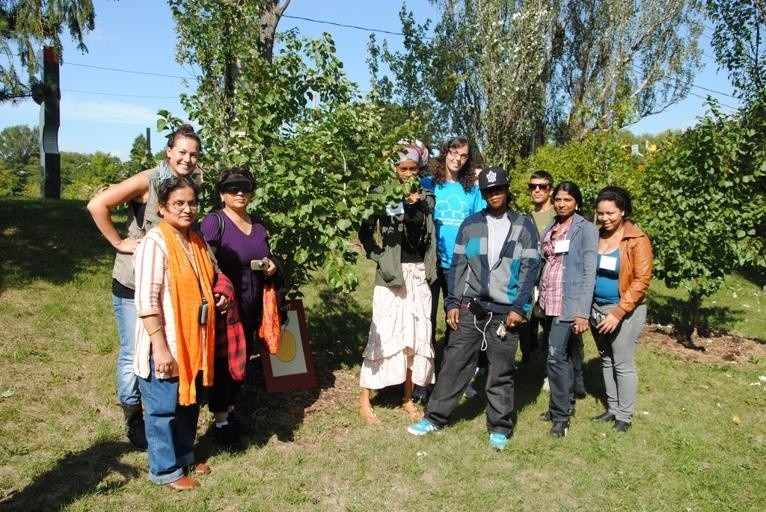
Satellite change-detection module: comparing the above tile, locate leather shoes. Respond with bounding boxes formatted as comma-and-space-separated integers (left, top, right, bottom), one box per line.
185, 462, 212, 475
591, 412, 615, 422
166, 476, 201, 491
611, 420, 631, 432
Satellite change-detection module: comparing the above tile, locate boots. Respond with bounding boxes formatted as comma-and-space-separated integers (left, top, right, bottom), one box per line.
121, 403, 149, 451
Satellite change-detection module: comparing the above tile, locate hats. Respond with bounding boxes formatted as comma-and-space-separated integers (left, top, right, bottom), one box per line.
479, 166, 508, 190
448, 150, 469, 159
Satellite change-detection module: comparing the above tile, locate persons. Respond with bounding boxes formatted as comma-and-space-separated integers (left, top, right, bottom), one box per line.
518, 169, 558, 395
199, 168, 276, 440
405, 167, 541, 451
538, 182, 598, 437
411, 135, 486, 399
357, 138, 436, 427
87, 125, 201, 451
131, 174, 229, 492
590, 186, 653, 432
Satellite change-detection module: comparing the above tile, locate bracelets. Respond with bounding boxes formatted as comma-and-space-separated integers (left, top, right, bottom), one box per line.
149, 325, 163, 336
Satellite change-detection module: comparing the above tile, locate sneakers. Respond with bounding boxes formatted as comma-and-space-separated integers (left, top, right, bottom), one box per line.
550, 422, 570, 439
215, 424, 242, 453
489, 433, 507, 449
408, 419, 442, 436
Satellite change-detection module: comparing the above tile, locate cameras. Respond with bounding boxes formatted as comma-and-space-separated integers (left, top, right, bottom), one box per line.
250, 260, 269, 270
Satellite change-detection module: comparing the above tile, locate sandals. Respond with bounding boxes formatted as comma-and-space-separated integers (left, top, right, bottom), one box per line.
359, 405, 381, 425
402, 404, 422, 419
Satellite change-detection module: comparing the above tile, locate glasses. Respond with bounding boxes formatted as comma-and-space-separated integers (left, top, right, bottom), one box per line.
221, 183, 253, 194
161, 202, 197, 210
528, 183, 551, 191
